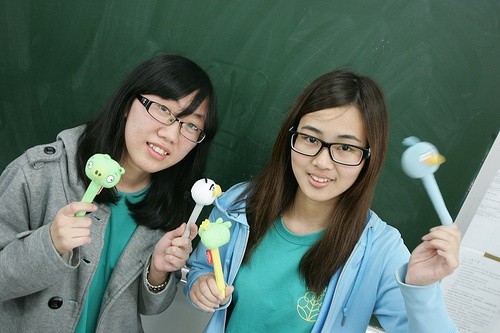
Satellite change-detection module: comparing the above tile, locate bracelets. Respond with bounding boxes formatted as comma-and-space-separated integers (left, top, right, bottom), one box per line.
146, 264, 170, 293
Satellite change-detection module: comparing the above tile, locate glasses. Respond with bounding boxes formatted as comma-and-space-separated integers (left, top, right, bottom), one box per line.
287, 124, 371, 167
131, 91, 206, 147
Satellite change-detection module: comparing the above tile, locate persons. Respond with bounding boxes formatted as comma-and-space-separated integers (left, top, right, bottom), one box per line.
183, 68, 460, 333
0, 55, 220, 333
